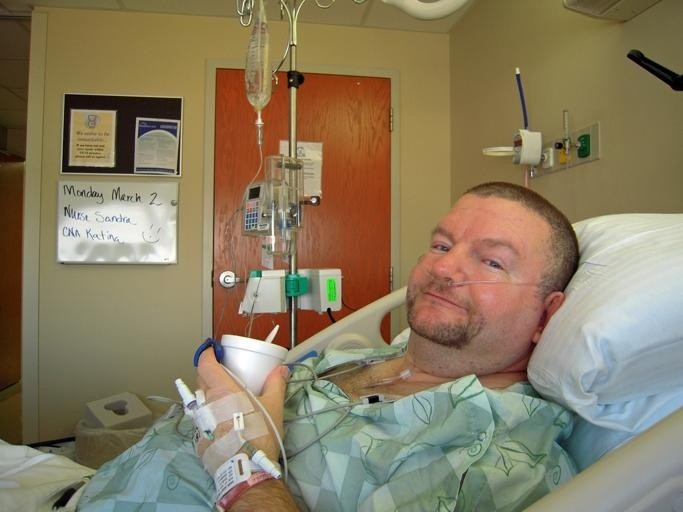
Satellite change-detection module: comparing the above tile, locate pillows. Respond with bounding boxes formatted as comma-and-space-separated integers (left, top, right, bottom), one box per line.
526, 212, 683, 431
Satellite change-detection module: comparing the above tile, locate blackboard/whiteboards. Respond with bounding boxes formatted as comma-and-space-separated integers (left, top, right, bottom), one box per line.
54, 181, 179, 267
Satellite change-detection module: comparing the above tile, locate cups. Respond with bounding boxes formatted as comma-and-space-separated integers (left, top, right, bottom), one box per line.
221, 334, 287, 398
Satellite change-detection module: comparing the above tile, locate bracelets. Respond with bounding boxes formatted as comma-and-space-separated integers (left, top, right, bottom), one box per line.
215, 452, 282, 512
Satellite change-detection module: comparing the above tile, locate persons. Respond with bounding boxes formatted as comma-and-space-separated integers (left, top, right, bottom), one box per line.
75, 182, 579, 512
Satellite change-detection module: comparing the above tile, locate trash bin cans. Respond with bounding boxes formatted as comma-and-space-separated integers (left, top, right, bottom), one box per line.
75, 418, 151, 469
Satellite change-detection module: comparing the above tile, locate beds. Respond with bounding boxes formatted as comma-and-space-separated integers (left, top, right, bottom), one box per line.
1, 213, 683, 512
